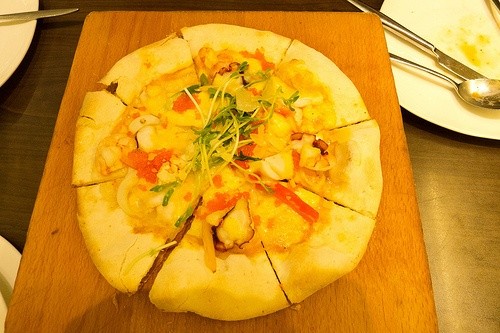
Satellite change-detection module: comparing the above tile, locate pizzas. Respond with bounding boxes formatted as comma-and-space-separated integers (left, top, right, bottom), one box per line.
72, 23, 384, 320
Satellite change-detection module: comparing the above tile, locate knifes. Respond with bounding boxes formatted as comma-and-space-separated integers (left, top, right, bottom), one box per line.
347, 0, 487, 81
0, 8, 80, 26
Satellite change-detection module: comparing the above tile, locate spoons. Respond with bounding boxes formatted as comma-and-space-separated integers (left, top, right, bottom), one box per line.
388, 52, 500, 109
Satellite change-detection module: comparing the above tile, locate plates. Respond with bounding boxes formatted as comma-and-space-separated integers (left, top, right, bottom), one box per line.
0, 0, 39, 89
1, 236, 23, 333
378, 0, 500, 139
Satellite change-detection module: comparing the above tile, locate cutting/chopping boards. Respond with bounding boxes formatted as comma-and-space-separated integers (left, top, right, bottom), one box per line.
2, 11, 439, 333
